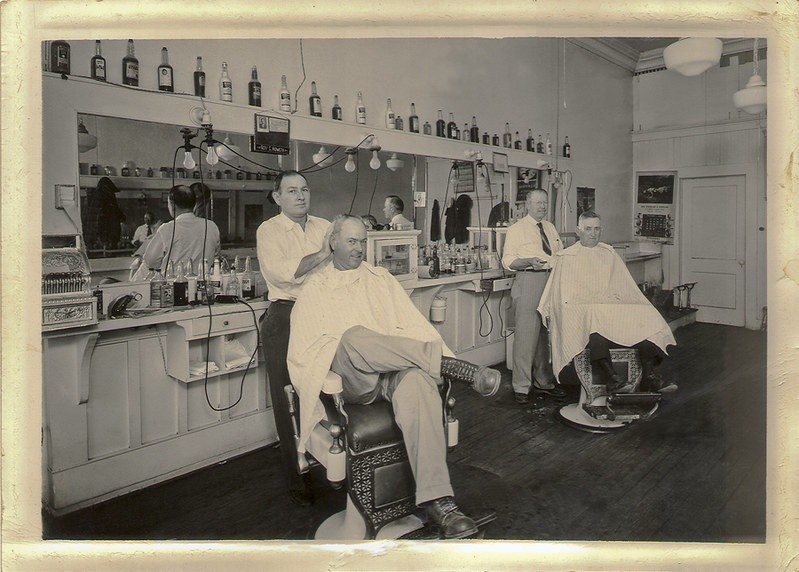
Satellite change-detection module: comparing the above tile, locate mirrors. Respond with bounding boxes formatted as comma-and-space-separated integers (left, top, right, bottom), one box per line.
77, 111, 549, 287
80, 188, 282, 256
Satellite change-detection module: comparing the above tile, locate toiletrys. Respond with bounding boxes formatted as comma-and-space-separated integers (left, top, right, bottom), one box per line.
418, 244, 484, 276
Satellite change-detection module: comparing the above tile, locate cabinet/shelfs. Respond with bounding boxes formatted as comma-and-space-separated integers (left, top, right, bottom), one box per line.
493, 227, 507, 259
466, 227, 493, 253
366, 226, 422, 284
165, 309, 261, 383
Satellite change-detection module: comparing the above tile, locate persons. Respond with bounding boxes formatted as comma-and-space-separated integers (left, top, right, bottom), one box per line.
130, 186, 223, 288
557, 211, 680, 395
289, 214, 501, 540
362, 195, 410, 230
500, 186, 569, 405
256, 169, 334, 512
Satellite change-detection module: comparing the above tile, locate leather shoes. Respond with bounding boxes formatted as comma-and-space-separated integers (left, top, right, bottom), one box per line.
514, 390, 529, 404
535, 384, 568, 397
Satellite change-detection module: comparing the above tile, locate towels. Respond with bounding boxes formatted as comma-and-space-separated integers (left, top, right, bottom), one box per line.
444, 200, 471, 245
431, 199, 441, 241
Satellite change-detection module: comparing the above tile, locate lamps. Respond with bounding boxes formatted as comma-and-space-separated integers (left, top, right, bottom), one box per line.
78, 118, 98, 156
731, 36, 768, 115
662, 35, 724, 77
215, 132, 241, 163
311, 143, 333, 170
385, 153, 404, 173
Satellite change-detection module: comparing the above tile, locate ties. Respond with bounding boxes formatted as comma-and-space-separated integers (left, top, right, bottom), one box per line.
536, 222, 552, 257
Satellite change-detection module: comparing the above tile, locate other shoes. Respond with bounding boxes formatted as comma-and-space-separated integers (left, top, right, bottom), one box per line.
607, 374, 635, 394
639, 372, 678, 394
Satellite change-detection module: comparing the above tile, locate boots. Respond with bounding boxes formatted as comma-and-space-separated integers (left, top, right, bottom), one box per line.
426, 498, 479, 539
441, 355, 502, 397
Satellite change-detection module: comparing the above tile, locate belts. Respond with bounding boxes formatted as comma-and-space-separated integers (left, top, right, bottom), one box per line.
277, 299, 295, 307
518, 268, 552, 273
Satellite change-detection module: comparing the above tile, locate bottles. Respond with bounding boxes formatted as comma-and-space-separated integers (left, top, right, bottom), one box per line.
122, 38, 139, 87
309, 81, 322, 117
151, 263, 255, 307
144, 255, 244, 280
436, 110, 460, 139
248, 65, 262, 107
218, 62, 232, 102
51, 40, 70, 75
463, 244, 489, 273
193, 56, 206, 97
332, 95, 342, 121
423, 122, 431, 134
527, 129, 552, 155
91, 40, 106, 81
493, 123, 522, 150
356, 92, 366, 124
90, 161, 278, 179
463, 116, 490, 144
418, 243, 440, 275
279, 73, 291, 112
408, 103, 419, 133
563, 136, 571, 158
157, 47, 174, 92
385, 98, 403, 131
440, 244, 465, 275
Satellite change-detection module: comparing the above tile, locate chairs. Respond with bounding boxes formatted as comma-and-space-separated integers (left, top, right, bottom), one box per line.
284, 371, 498, 539
546, 313, 664, 433
